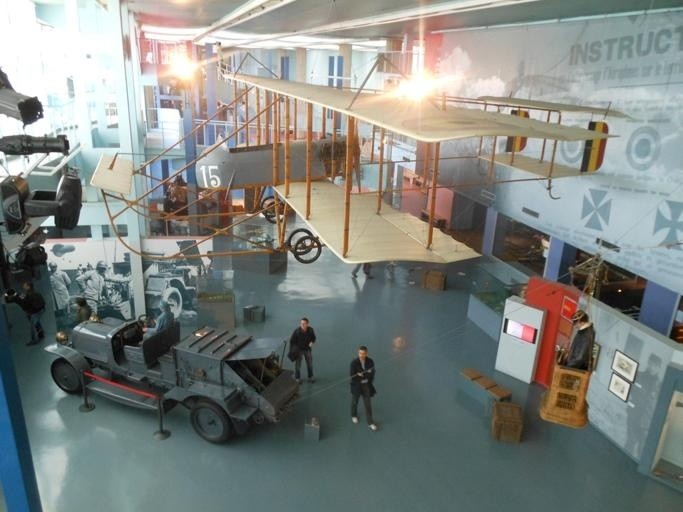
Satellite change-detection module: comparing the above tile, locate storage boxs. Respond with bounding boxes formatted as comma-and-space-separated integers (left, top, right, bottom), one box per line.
488, 400, 523, 445
421, 268, 449, 290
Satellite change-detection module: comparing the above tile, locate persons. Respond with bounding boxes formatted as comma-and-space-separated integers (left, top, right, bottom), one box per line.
75, 257, 106, 318
74, 297, 91, 322
46, 261, 73, 330
564, 310, 596, 371
347, 345, 379, 433
139, 300, 173, 345
287, 316, 317, 386
350, 262, 374, 280
10, 280, 46, 346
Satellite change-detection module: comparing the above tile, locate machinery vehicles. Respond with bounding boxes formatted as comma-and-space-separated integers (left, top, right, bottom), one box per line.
46, 305, 300, 445
74, 250, 205, 324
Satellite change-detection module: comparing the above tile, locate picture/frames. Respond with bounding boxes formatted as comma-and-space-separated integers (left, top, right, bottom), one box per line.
606, 372, 631, 403
610, 350, 638, 383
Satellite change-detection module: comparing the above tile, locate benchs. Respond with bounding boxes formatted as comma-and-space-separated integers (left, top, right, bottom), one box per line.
453, 368, 513, 412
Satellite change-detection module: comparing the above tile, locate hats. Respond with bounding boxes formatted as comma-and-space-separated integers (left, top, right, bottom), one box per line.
158, 301, 169, 308
571, 310, 584, 321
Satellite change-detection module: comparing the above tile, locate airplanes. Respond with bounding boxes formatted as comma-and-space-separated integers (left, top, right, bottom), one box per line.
90, 51, 629, 265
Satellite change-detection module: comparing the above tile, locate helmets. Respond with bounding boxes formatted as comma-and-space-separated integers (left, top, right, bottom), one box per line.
97, 261, 108, 269
49, 261, 57, 267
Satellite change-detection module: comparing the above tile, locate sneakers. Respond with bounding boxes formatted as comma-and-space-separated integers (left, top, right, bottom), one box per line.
369, 424, 377, 431
352, 417, 358, 423
27, 330, 44, 344
